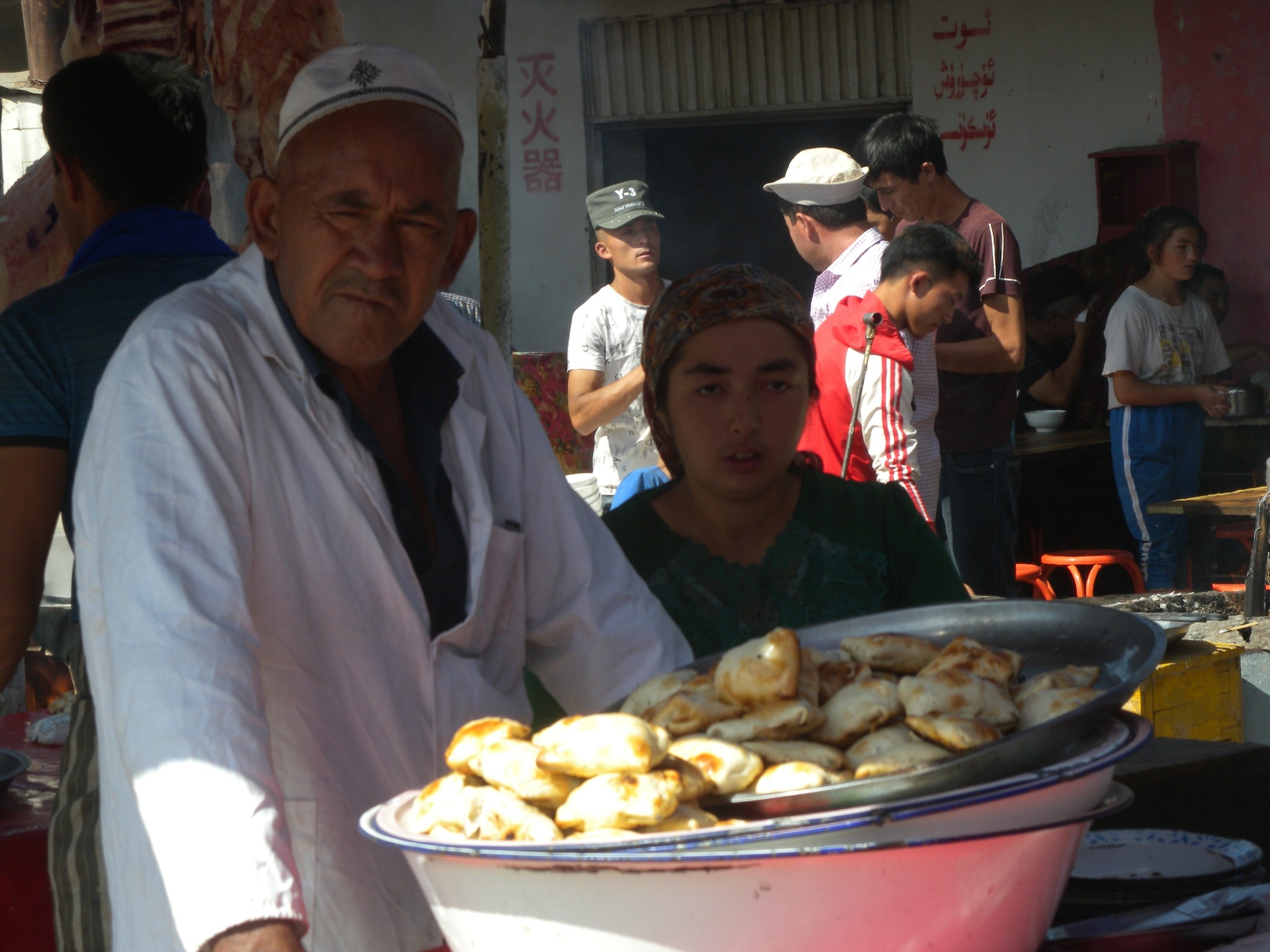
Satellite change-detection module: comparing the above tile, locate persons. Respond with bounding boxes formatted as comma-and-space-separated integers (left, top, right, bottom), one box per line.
753, 108, 1270, 595
0, 53, 241, 952
520, 265, 973, 736
566, 179, 675, 515
68, 41, 693, 952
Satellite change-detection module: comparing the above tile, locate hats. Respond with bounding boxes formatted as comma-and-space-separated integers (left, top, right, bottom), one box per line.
763, 146, 872, 208
272, 40, 464, 149
586, 178, 668, 230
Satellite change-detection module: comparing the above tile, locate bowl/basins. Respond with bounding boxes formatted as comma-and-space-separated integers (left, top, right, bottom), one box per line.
357, 709, 1153, 952
1024, 410, 1067, 432
1153, 619, 1196, 644
565, 473, 604, 517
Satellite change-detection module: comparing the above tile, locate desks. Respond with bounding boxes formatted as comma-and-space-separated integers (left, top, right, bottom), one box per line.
1147, 485, 1270, 516
0, 710, 65, 952
1122, 638, 1245, 743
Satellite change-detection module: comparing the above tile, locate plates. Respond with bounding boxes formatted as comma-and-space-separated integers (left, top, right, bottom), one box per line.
603, 595, 1168, 818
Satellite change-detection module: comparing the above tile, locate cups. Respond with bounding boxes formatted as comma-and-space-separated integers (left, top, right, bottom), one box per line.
1227, 389, 1247, 417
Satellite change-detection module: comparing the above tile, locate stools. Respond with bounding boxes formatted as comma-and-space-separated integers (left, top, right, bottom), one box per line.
1212, 583, 1270, 592
1016, 549, 1146, 601
1216, 520, 1255, 554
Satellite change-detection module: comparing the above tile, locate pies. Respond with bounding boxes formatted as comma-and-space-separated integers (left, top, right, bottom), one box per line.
404, 629, 1107, 841
